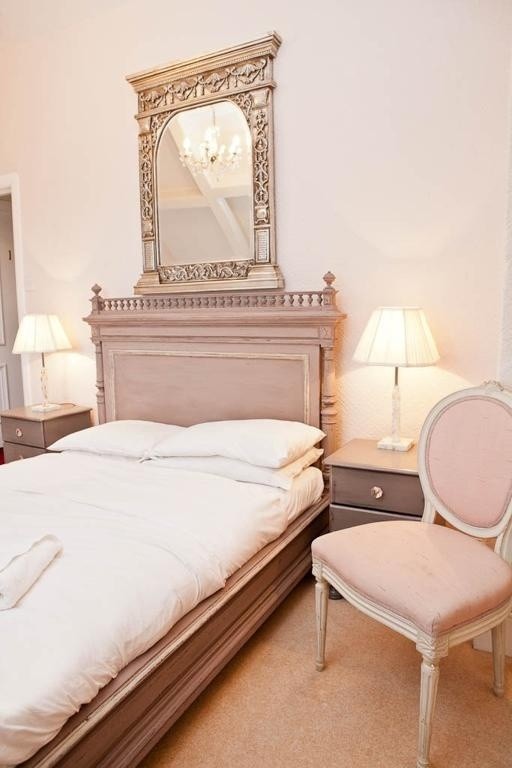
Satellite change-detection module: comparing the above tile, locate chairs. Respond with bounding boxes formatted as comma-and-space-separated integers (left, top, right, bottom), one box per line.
309, 377, 511, 768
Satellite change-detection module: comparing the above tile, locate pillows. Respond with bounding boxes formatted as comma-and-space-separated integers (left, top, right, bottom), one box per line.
49, 420, 321, 490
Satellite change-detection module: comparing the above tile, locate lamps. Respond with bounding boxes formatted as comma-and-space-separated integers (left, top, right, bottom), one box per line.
352, 307, 441, 453
175, 104, 247, 183
12, 313, 73, 414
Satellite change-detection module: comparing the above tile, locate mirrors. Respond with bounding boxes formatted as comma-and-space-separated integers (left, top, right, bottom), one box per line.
122, 31, 284, 290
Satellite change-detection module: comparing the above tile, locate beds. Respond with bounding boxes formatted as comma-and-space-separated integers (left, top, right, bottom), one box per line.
0, 453, 330, 768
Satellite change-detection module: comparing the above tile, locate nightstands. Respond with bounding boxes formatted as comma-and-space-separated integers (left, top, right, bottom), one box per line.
324, 438, 443, 604
0, 406, 88, 459
